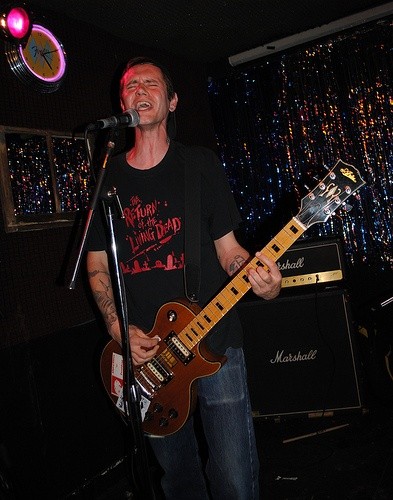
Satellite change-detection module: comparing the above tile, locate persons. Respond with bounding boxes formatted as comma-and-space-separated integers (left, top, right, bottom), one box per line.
76, 57, 282, 499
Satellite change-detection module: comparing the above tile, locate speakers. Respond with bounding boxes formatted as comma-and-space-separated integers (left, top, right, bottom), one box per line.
239, 286, 368, 417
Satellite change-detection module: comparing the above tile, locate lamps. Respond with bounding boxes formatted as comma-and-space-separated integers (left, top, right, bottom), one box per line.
0, 4, 33, 44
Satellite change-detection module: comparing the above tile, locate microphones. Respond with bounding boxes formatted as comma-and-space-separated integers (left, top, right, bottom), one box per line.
87, 107, 141, 131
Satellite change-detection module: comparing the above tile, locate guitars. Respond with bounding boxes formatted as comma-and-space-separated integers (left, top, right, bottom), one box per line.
101, 158, 366, 437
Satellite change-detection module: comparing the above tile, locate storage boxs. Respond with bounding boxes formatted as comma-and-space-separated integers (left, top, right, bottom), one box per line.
241, 285, 363, 424
